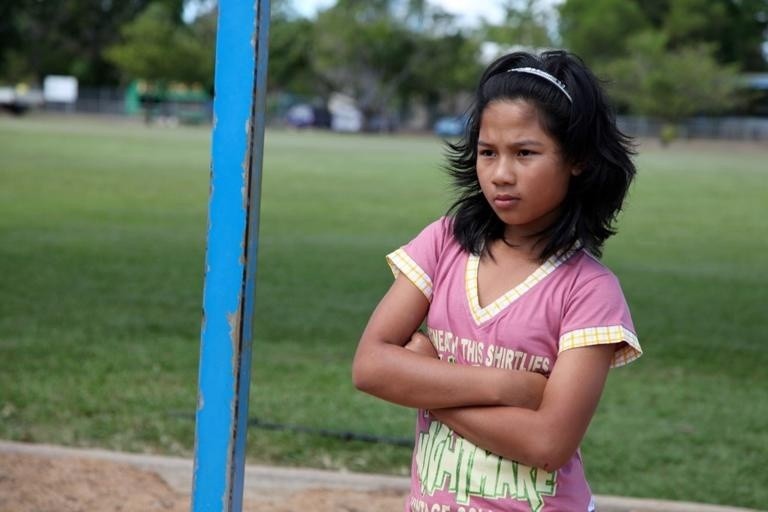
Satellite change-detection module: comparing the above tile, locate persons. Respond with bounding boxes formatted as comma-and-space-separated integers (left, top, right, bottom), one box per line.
352, 52, 644, 512
